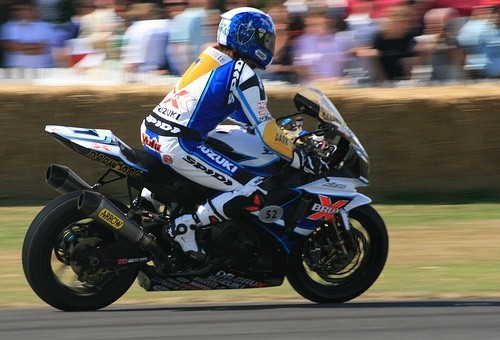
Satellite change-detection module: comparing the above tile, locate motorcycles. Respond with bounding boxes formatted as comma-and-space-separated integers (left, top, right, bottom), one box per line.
21, 86, 390, 312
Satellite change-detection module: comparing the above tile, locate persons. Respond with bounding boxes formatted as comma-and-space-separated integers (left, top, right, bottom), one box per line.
138, 6, 338, 264
0, 1, 500, 88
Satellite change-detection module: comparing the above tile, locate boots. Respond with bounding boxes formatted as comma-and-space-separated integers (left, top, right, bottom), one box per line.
162, 198, 230, 262
133, 187, 165, 215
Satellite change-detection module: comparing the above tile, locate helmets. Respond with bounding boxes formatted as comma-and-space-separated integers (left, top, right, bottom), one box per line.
217, 7, 276, 70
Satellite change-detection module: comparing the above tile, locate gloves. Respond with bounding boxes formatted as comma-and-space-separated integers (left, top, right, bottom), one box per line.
290, 147, 333, 177
